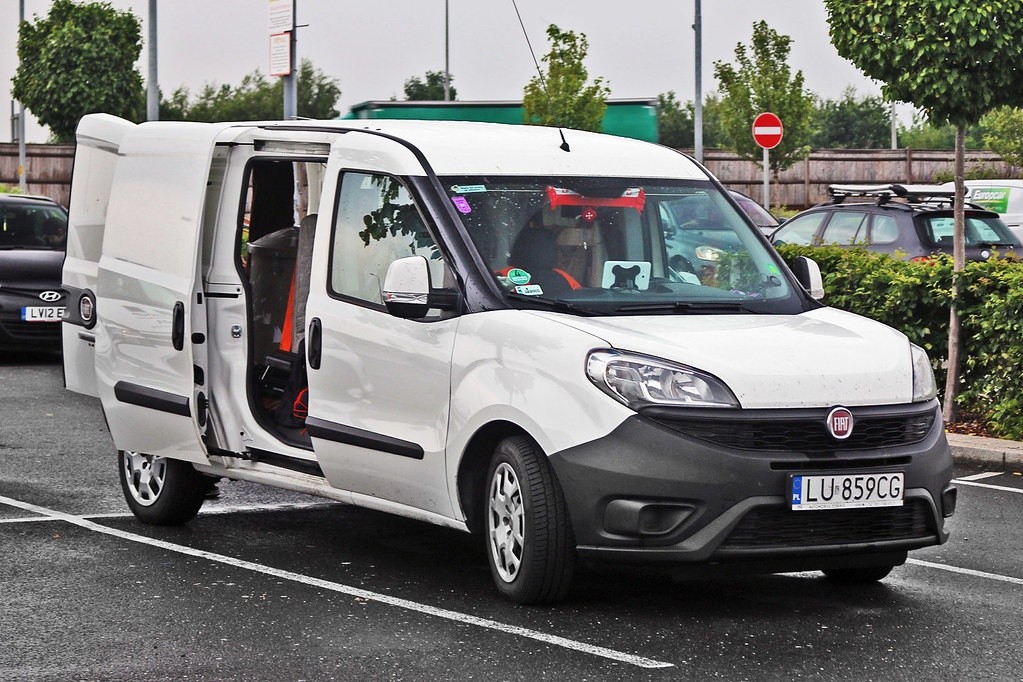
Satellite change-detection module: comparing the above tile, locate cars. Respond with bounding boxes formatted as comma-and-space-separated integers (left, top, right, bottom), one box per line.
244, 183, 300, 228
1, 193, 70, 358
660, 190, 788, 278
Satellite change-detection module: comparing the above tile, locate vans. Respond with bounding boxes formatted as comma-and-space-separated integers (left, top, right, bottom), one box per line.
919, 179, 1023, 246
59, 117, 957, 607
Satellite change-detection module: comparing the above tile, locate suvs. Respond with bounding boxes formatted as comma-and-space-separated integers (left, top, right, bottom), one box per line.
765, 182, 1023, 267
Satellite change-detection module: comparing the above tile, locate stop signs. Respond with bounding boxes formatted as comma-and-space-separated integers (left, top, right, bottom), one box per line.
753, 113, 783, 150
